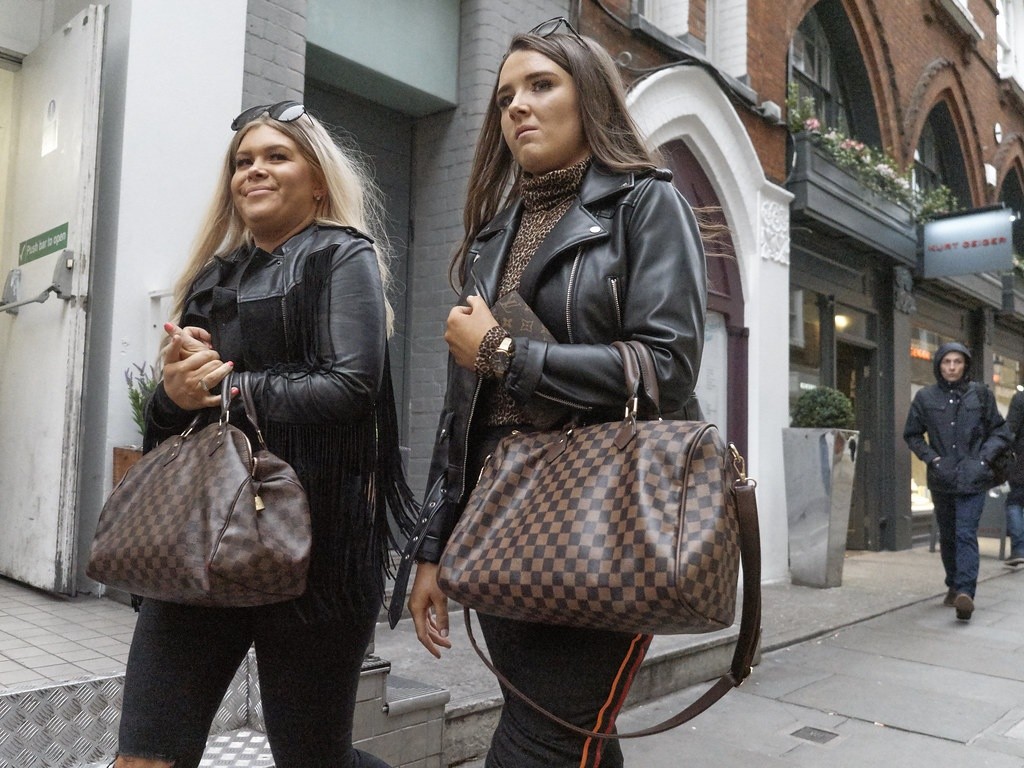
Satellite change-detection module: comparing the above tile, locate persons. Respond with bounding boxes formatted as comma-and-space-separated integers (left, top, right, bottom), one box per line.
903, 343, 1024, 621
114, 101, 419, 768
388, 14, 708, 768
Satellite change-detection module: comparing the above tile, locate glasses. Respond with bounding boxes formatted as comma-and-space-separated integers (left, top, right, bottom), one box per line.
502, 16, 593, 58
231, 101, 315, 131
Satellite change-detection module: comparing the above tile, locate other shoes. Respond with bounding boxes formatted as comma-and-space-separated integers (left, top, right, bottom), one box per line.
944, 588, 958, 606
1004, 553, 1024, 567
956, 591, 974, 619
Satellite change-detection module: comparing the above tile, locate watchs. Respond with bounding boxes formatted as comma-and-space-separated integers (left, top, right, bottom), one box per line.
489, 337, 514, 380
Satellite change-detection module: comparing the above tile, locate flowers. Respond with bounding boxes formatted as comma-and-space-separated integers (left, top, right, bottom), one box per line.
786, 85, 922, 207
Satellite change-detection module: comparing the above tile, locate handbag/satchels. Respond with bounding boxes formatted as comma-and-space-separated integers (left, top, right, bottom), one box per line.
436, 340, 741, 635
85, 370, 312, 607
489, 290, 571, 432
975, 382, 1017, 490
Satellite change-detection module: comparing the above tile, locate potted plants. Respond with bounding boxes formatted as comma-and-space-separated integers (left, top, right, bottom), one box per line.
783, 385, 861, 590
112, 358, 163, 609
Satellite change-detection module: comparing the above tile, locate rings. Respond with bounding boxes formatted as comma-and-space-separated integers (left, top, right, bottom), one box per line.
198, 378, 209, 392
